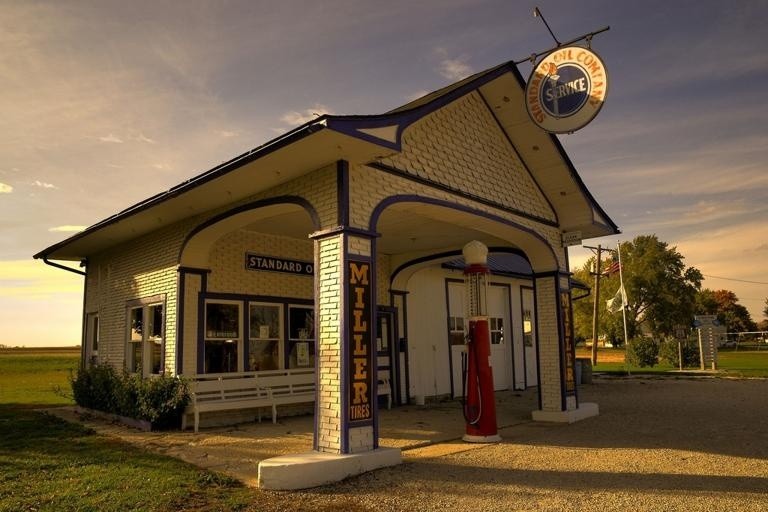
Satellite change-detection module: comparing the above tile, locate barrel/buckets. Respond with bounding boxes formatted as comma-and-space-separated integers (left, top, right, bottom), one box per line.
415, 394, 425, 405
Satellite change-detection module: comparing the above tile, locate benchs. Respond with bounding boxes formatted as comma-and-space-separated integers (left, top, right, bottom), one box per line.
183, 367, 392, 435
